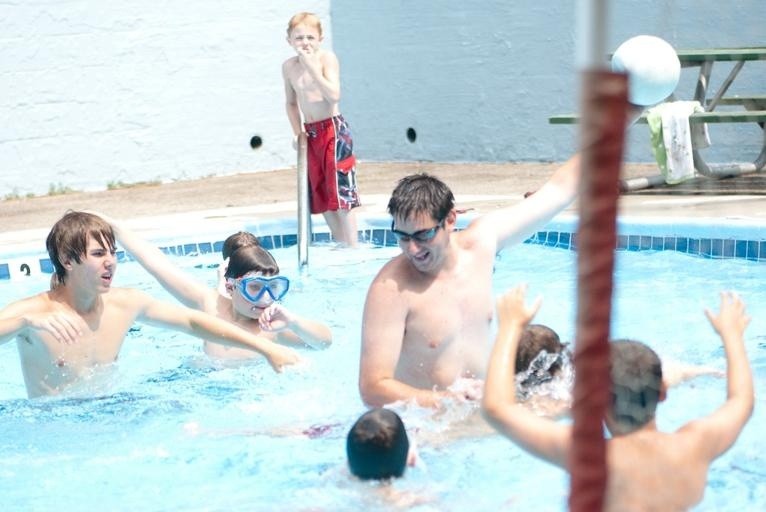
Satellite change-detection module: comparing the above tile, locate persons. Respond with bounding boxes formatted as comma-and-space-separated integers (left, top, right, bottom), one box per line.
281, 11, 364, 248
0, 210, 310, 403
343, 405, 442, 510
70, 208, 334, 362
421, 316, 730, 454
357, 151, 582, 424
481, 278, 758, 512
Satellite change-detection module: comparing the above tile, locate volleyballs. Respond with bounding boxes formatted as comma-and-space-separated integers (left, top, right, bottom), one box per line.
611, 35, 681, 107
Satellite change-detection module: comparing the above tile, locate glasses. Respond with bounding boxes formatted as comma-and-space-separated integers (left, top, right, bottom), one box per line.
227, 275, 290, 304
390, 217, 446, 242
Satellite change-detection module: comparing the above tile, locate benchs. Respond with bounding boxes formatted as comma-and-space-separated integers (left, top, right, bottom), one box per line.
549, 46, 766, 193
